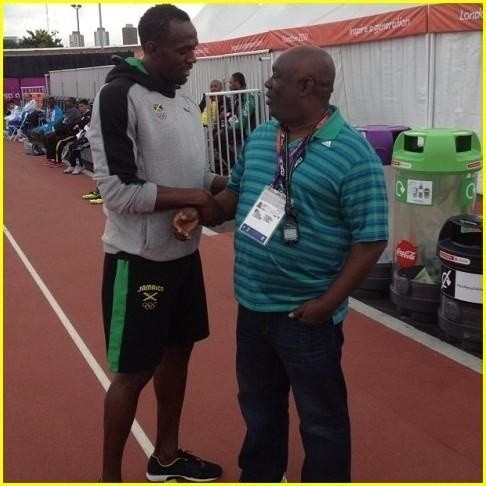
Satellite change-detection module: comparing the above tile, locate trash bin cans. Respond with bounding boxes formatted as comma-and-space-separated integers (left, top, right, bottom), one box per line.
352, 125, 483, 343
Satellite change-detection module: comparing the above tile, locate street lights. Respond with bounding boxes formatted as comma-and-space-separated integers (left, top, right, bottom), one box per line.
72, 4, 83, 46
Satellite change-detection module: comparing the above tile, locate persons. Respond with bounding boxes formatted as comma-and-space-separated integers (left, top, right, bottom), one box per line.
3, 93, 103, 206
212, 72, 256, 166
89, 3, 227, 481
171, 44, 390, 481
199, 79, 229, 135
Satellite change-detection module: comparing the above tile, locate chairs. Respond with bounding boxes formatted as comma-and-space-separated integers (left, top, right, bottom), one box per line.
5, 110, 29, 142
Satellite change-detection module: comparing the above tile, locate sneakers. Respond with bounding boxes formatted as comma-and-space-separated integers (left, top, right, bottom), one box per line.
82, 191, 99, 198
145, 448, 222, 482
63, 166, 75, 173
87, 197, 103, 203
32, 144, 44, 155
72, 164, 85, 174
